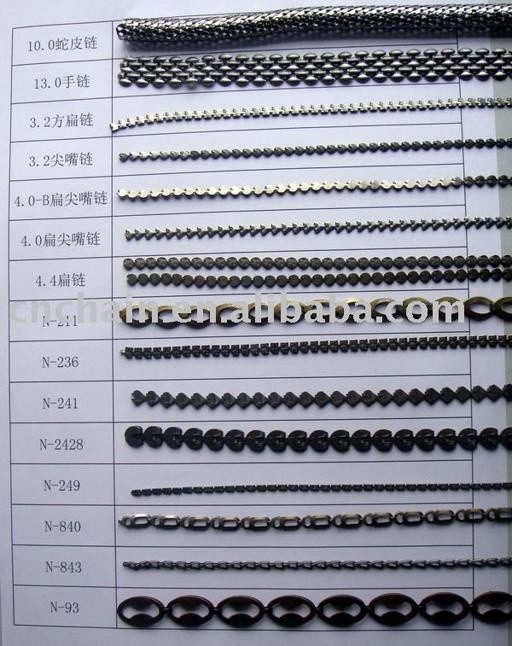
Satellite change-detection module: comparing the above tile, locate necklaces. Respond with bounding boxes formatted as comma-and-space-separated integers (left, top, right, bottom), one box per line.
111, 3, 511, 629
